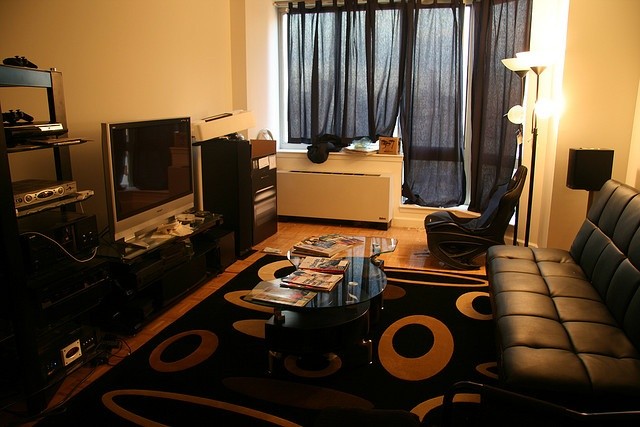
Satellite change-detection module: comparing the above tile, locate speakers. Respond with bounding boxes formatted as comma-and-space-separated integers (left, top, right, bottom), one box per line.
200, 137, 254, 257
565, 146, 615, 192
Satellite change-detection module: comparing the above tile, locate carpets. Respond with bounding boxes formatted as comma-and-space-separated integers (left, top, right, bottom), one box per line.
38, 254, 502, 426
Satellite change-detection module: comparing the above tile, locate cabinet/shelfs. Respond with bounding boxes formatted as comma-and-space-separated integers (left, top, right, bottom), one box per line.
123, 206, 234, 336
0, 64, 110, 417
277, 144, 406, 230
201, 138, 277, 249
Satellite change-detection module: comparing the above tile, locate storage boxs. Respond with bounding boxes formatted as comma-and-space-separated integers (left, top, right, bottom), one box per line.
378, 137, 400, 154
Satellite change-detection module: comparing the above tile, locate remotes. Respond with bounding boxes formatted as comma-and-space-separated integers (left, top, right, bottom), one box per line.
319, 259, 340, 268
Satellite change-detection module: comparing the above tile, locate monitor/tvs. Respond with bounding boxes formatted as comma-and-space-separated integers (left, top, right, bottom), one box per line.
101, 116, 195, 249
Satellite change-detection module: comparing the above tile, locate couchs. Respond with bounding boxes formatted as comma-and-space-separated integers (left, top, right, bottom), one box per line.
441, 180, 640, 427
424, 167, 527, 268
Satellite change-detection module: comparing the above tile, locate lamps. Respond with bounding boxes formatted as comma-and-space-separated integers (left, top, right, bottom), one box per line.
502, 45, 556, 246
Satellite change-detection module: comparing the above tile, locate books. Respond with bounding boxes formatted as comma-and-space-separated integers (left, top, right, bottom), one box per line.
279, 271, 343, 292
299, 255, 350, 274
292, 234, 364, 258
243, 280, 318, 306
282, 269, 341, 289
